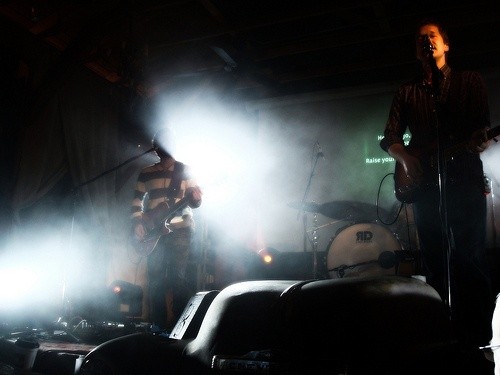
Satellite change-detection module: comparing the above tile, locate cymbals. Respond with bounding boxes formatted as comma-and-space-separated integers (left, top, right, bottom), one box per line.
316, 200, 390, 222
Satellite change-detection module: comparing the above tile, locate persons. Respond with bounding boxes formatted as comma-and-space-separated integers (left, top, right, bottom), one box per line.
128, 127, 202, 334
379, 20, 495, 375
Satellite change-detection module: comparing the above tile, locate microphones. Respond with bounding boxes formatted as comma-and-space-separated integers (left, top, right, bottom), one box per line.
424, 35, 433, 53
315, 143, 325, 160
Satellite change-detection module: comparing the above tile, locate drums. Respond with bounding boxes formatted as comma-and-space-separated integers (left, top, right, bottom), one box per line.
308, 219, 356, 278
391, 203, 416, 227
271, 251, 325, 279
326, 223, 405, 280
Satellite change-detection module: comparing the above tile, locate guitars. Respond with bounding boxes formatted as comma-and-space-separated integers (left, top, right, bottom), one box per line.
131, 185, 204, 257
394, 124, 500, 202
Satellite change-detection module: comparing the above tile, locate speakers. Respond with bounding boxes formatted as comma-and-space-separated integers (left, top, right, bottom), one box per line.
168, 278, 486, 375
